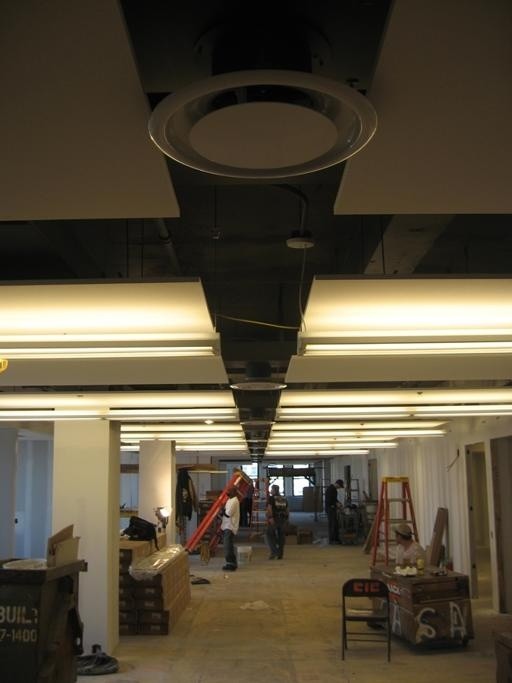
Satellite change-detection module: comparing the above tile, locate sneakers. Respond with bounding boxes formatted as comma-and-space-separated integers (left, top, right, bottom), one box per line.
223, 564, 237, 570
270, 551, 283, 559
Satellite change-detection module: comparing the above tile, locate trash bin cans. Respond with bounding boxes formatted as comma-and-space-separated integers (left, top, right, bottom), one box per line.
1, 558, 85, 683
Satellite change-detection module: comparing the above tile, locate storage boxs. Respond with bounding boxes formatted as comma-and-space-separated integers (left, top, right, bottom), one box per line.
118, 532, 192, 636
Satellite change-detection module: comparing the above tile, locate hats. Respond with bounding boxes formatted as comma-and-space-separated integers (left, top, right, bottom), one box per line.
392, 525, 412, 537
336, 479, 344, 488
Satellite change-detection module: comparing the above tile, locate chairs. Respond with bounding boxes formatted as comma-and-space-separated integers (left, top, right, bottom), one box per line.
341, 578, 391, 662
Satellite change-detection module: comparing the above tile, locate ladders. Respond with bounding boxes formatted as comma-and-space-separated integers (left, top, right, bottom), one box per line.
372, 477, 419, 566
184, 469, 253, 555
314, 461, 360, 521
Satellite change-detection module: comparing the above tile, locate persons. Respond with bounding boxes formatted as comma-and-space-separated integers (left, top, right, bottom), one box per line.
217, 478, 254, 571
325, 479, 346, 545
392, 523, 426, 567
264, 485, 290, 560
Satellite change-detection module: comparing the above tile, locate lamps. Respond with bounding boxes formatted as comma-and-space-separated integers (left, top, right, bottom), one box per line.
159, 508, 172, 524
